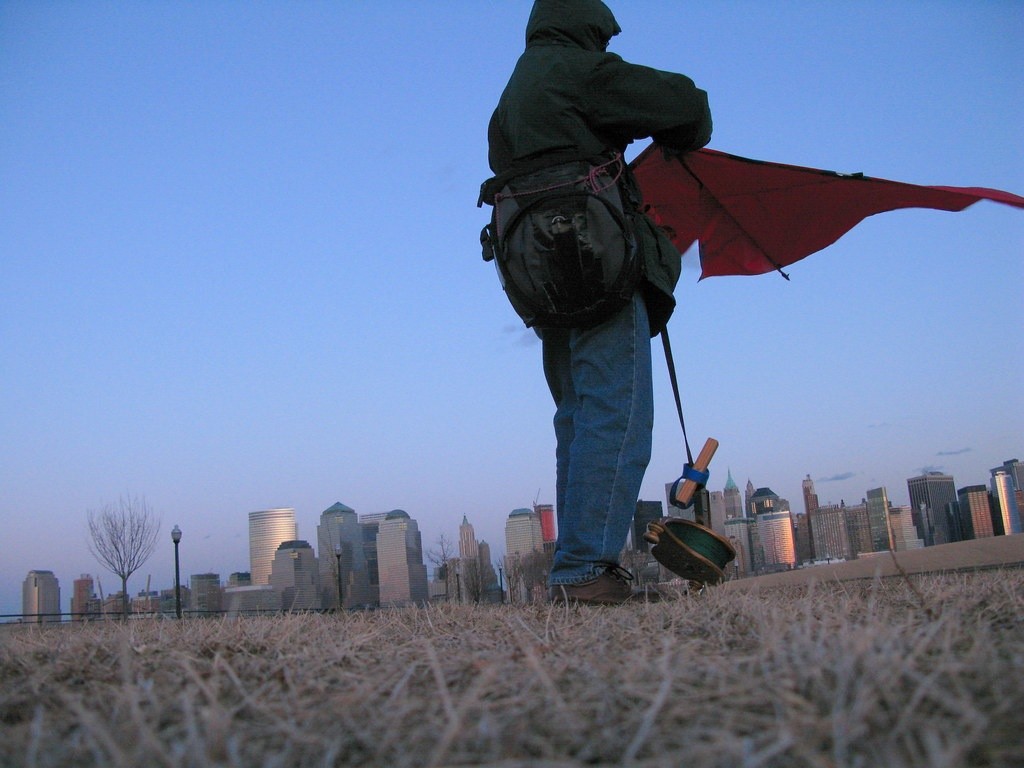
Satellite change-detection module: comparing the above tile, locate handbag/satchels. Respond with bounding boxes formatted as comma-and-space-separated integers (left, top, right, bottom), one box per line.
478, 143, 639, 329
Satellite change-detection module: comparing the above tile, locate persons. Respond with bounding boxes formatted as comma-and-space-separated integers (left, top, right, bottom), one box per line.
488, 0, 714, 606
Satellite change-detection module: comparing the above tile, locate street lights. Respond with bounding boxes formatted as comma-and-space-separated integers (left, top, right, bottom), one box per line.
454, 565, 461, 602
170, 524, 182, 620
506, 571, 513, 601
542, 568, 548, 590
728, 534, 741, 581
498, 563, 504, 605
333, 543, 344, 613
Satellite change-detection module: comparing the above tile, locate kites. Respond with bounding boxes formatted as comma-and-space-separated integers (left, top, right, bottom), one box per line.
623, 142, 1024, 284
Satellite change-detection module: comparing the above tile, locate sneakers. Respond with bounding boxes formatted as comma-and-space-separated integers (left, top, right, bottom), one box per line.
548, 564, 672, 609
